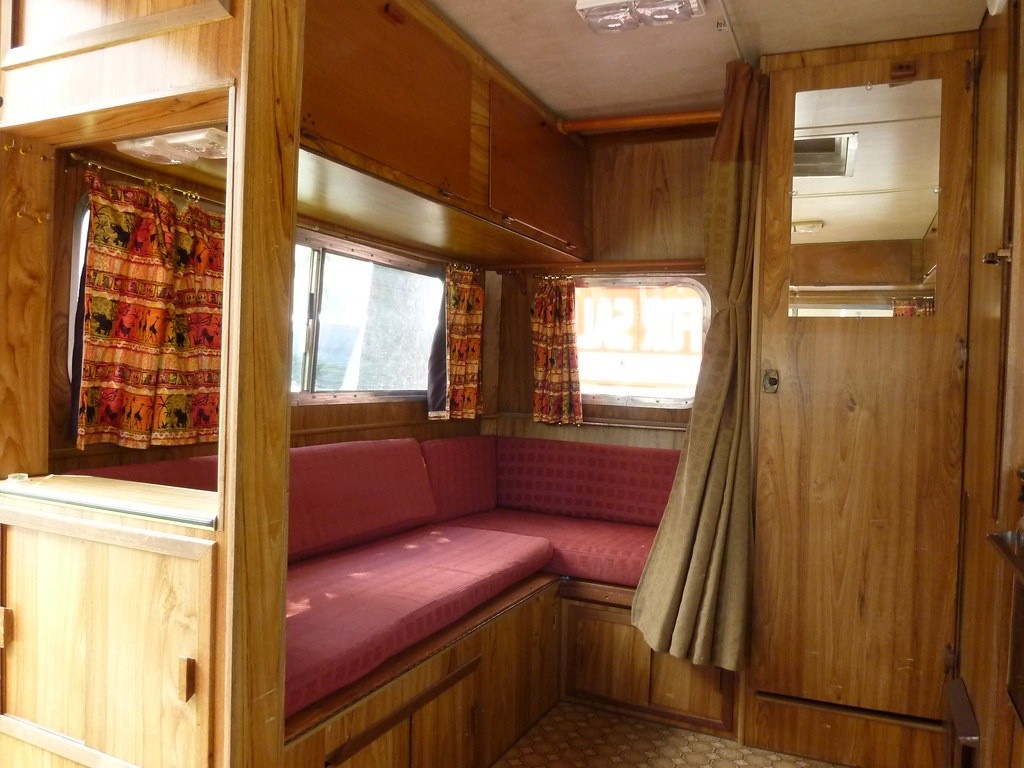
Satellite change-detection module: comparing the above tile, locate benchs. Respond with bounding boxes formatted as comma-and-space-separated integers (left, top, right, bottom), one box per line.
63, 436, 551, 722
423, 433, 681, 588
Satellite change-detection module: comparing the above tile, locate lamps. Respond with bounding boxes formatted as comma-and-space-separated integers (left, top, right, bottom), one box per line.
575, 0, 706, 35
791, 221, 824, 233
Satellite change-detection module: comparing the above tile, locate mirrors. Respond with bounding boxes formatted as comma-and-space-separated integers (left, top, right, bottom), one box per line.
788, 78, 944, 319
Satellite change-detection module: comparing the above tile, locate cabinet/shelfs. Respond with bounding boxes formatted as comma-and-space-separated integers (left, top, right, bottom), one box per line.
559, 598, 738, 741
319, 655, 483, 768
